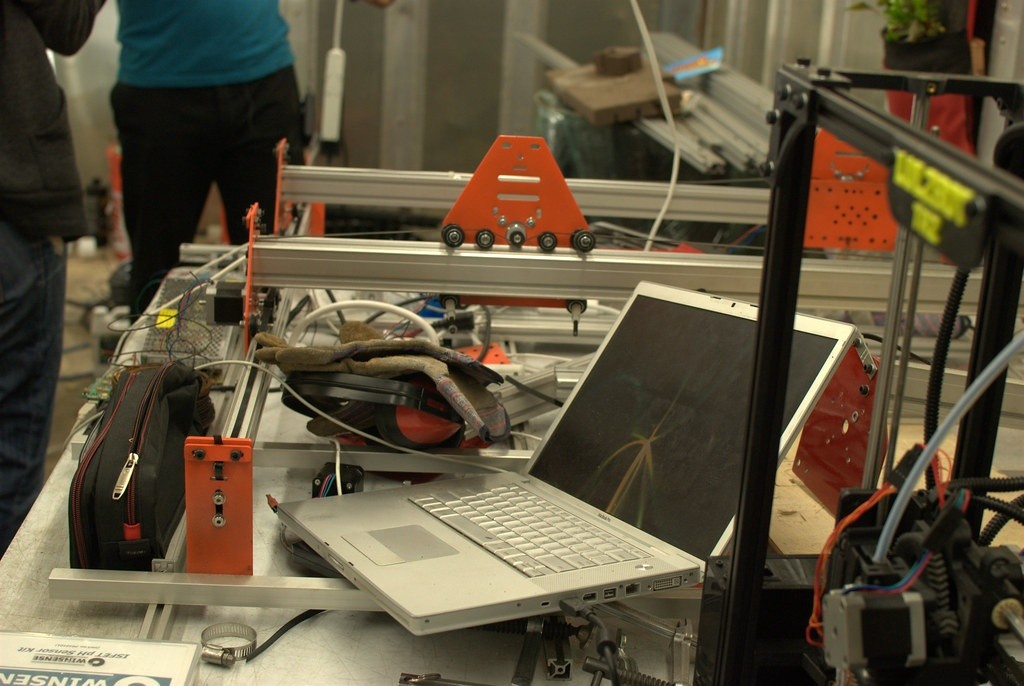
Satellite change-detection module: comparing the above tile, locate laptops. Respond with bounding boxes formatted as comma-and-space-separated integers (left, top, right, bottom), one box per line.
279, 282, 861, 635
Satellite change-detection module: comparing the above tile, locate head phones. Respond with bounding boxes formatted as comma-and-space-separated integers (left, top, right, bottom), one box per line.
284, 368, 467, 451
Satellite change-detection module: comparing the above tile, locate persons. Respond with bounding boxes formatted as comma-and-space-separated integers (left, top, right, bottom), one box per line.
0, 0, 108, 559
109, 1, 396, 324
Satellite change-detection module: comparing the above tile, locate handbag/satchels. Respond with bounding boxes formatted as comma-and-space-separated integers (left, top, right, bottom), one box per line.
67, 360, 216, 572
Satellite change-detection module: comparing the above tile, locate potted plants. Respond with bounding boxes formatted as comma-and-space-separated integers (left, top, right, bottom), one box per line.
848, 0, 971, 71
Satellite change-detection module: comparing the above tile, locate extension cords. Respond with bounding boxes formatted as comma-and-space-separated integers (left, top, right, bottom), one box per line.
320, 48, 347, 142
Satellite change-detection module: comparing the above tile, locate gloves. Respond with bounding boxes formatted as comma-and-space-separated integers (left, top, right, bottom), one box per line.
254, 319, 510, 441
